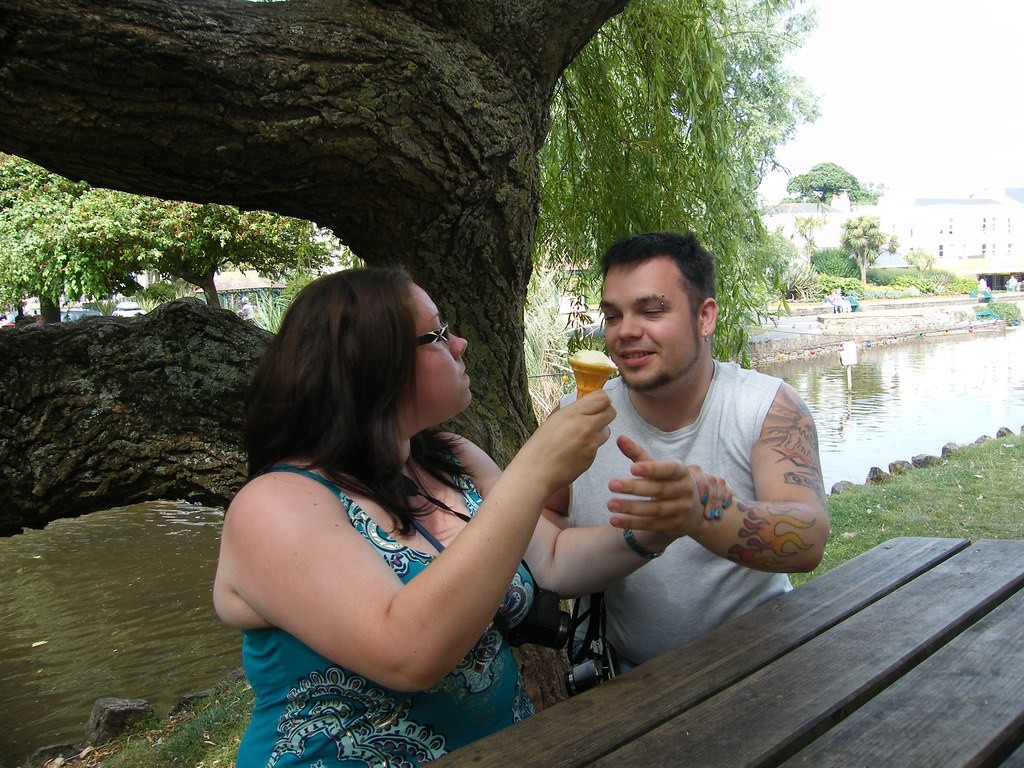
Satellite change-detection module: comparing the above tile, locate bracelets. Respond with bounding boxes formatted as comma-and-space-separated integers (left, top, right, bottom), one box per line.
623, 529, 666, 559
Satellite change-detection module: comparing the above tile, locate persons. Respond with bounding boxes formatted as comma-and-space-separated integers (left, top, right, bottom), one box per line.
212, 265, 734, 768
827, 289, 861, 313
23, 315, 44, 329
242, 296, 253, 323
7, 313, 15, 323
539, 232, 831, 675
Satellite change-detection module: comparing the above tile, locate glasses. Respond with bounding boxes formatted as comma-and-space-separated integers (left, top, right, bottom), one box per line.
413, 320, 449, 345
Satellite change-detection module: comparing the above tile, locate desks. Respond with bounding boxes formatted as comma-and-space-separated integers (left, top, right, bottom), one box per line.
420, 535, 1024, 768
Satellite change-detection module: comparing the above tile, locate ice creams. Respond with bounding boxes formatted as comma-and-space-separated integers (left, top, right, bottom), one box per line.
569, 349, 618, 399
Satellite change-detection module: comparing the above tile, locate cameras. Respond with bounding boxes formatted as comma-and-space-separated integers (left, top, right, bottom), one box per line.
500, 586, 571, 650
562, 636, 621, 698
